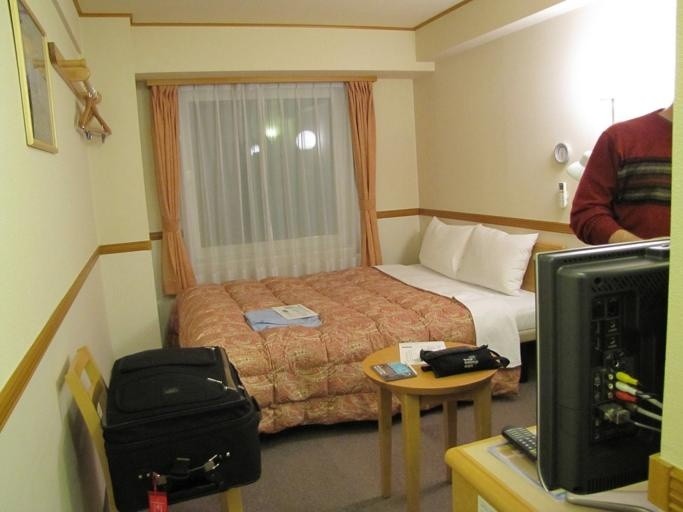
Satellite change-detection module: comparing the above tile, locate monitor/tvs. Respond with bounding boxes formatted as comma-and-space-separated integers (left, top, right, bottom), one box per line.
535, 237, 671, 494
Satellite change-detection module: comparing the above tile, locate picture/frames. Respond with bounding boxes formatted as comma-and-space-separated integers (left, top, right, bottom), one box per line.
5, 1, 59, 157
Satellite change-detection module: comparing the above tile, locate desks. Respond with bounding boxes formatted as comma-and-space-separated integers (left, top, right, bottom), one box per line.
358, 338, 501, 511
442, 426, 647, 512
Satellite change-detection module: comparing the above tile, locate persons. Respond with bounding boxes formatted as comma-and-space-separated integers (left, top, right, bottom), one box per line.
567, 91, 675, 244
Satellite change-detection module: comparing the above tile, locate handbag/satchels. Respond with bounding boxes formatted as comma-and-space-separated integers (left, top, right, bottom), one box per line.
421, 345, 506, 379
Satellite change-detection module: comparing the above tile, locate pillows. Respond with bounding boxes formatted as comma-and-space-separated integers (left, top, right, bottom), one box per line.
417, 214, 478, 278
453, 224, 539, 297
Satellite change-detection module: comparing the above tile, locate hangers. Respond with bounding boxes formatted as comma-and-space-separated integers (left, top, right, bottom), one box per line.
76, 88, 114, 144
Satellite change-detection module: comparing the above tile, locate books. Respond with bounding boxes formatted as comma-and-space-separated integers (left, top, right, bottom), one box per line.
369, 360, 418, 383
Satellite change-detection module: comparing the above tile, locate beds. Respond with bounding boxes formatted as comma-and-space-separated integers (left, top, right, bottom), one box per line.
171, 237, 567, 438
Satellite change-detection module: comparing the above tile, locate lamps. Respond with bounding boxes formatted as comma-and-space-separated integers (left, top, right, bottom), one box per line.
565, 146, 597, 186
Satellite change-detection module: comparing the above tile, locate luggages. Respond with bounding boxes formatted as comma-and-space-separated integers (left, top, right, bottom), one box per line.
103, 345, 261, 512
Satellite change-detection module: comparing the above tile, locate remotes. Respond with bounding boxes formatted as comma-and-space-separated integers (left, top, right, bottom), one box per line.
501, 425, 537, 462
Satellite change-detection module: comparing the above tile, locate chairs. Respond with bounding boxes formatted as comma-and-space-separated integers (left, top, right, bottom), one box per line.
64, 344, 247, 512
